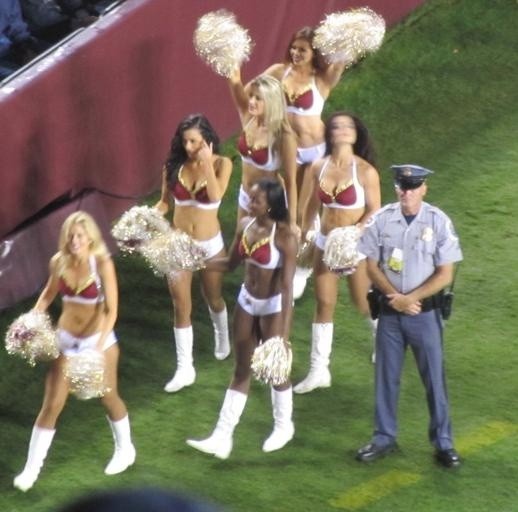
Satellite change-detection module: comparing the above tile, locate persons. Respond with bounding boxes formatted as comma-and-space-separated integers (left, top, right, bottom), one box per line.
356, 164, 464, 470
3, 209, 136, 492
0, 0, 112, 82
140, 175, 296, 460
265, 4, 387, 195
293, 112, 381, 393
109, 111, 232, 393
190, 8, 302, 238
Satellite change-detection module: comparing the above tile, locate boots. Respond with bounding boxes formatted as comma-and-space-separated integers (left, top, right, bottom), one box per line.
262, 384, 294, 452
294, 267, 313, 301
367, 317, 379, 364
104, 414, 135, 475
165, 326, 195, 392
13, 425, 56, 492
208, 304, 230, 361
185, 388, 248, 460
294, 323, 334, 394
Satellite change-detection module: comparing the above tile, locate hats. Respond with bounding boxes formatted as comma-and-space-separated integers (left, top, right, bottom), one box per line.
390, 165, 434, 191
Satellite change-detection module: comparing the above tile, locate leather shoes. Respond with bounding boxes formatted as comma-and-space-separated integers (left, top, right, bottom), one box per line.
357, 443, 398, 461
440, 449, 463, 466
